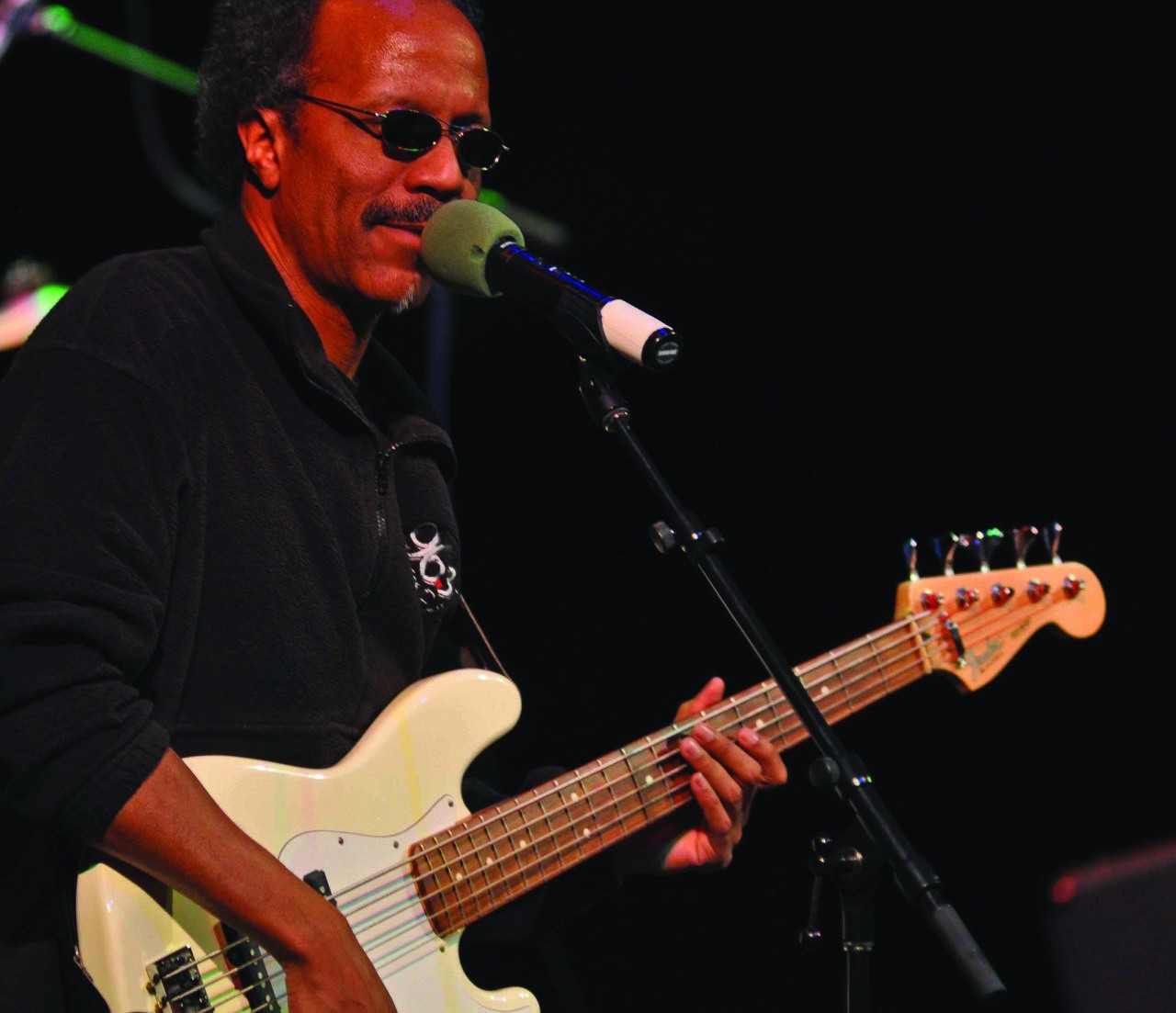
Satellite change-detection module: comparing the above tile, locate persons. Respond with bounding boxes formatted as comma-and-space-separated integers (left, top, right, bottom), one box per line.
0, 0, 789, 1013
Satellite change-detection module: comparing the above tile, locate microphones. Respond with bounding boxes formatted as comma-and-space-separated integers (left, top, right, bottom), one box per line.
419, 199, 682, 372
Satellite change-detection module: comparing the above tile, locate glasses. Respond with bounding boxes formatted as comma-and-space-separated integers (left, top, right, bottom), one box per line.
295, 93, 509, 171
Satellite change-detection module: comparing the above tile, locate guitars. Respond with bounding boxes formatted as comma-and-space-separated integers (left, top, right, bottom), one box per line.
69, 522, 1108, 1013
